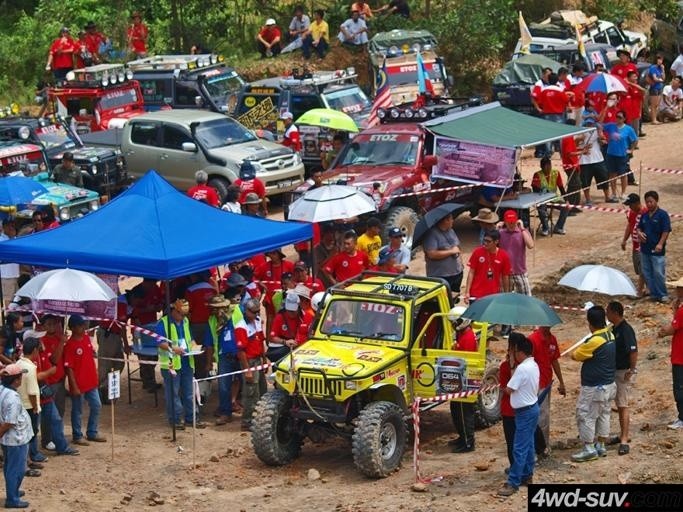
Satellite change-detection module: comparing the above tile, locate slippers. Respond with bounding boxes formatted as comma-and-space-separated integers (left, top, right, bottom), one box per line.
605, 198, 618, 203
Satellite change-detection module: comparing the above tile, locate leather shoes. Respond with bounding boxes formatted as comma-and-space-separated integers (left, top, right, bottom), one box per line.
26, 432, 107, 477
498, 445, 550, 496
453, 442, 475, 453
17, 490, 25, 496
6, 501, 28, 510
447, 438, 464, 446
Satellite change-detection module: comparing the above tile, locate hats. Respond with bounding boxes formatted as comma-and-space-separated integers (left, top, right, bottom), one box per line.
389, 226, 406, 238
205, 249, 312, 312
23, 328, 48, 342
265, 17, 276, 26
623, 193, 640, 205
68, 315, 84, 325
277, 111, 294, 120
126, 287, 144, 297
367, 217, 385, 227
503, 210, 518, 223
63, 152, 73, 159
471, 208, 499, 224
664, 276, 683, 287
378, 246, 401, 265
2, 363, 30, 376
240, 160, 257, 180
241, 192, 263, 205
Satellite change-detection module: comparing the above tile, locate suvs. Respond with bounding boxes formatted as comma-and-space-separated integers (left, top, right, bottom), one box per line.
492, 41, 664, 124
367, 29, 450, 110
280, 94, 483, 264
249, 269, 507, 475
510, 8, 650, 75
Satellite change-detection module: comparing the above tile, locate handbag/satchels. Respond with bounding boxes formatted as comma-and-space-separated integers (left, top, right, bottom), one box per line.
39, 385, 54, 399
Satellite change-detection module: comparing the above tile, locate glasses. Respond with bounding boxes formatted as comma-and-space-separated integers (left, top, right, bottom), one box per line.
248, 309, 260, 314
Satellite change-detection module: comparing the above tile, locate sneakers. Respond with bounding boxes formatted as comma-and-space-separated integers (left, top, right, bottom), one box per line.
569, 211, 576, 215
620, 194, 629, 200
666, 418, 682, 430
585, 200, 592, 205
571, 435, 631, 462
554, 227, 566, 234
571, 207, 582, 212
542, 225, 550, 236
629, 181, 639, 186
173, 401, 242, 431
612, 195, 618, 199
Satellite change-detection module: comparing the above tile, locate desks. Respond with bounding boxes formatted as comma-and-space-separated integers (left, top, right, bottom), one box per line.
494, 192, 559, 239
